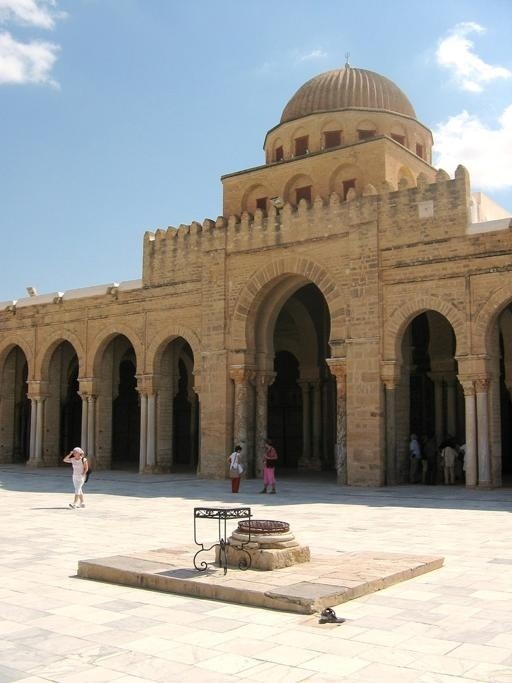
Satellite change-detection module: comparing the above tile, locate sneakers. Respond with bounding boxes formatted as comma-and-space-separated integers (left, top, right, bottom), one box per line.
69, 504, 77, 508
260, 489, 266, 493
270, 490, 276, 493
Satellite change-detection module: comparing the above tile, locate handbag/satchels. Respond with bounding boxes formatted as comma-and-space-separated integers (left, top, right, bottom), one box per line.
230, 467, 239, 478
81, 458, 91, 482
266, 459, 275, 467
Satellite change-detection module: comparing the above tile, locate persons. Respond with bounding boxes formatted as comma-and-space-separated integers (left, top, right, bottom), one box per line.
62, 446, 89, 508
410, 434, 421, 484
460, 443, 468, 479
422, 437, 432, 484
259, 438, 278, 493
226, 444, 242, 493
440, 442, 459, 483
